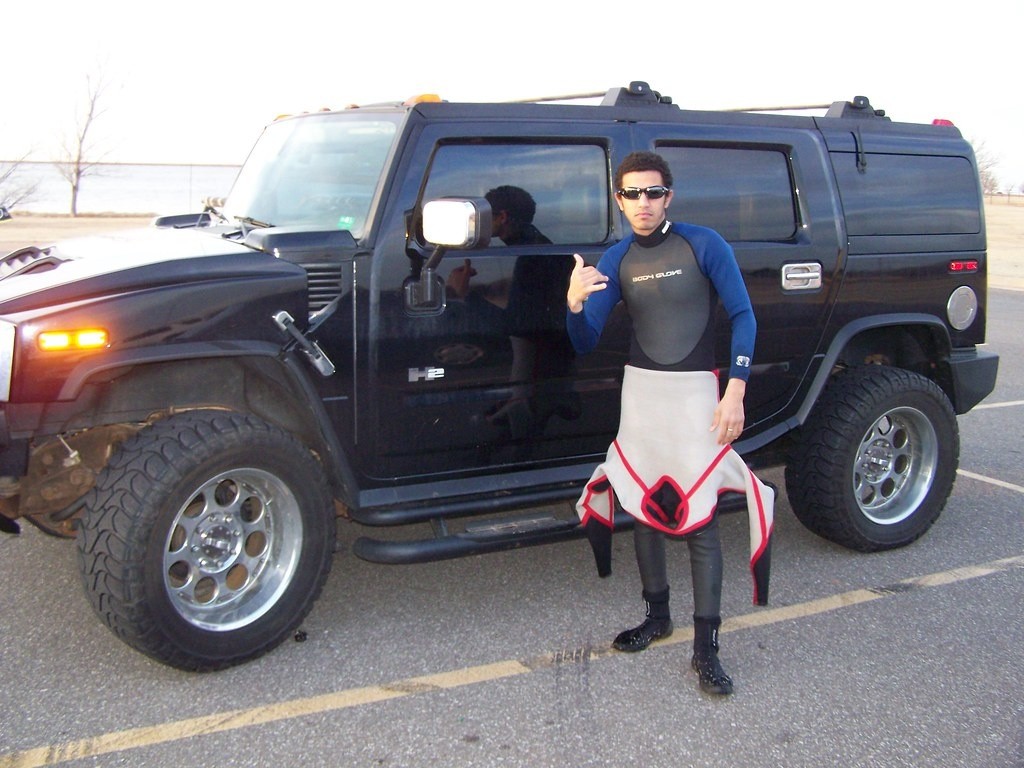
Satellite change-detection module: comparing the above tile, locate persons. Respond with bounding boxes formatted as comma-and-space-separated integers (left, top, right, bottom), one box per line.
448, 185, 577, 464
567, 152, 757, 696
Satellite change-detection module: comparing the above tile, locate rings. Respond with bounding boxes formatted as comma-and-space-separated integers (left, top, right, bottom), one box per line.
727, 428, 733, 432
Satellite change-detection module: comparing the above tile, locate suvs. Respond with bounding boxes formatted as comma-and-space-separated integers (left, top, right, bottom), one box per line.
0, 81, 1001, 674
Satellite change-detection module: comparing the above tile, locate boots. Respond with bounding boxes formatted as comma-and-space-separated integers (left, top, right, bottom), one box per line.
692, 613, 734, 694
612, 583, 673, 652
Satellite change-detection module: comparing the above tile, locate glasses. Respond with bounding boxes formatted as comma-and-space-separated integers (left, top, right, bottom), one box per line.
618, 185, 670, 199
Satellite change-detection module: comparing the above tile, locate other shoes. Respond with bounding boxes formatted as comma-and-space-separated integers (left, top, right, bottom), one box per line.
0, 514, 20, 534
